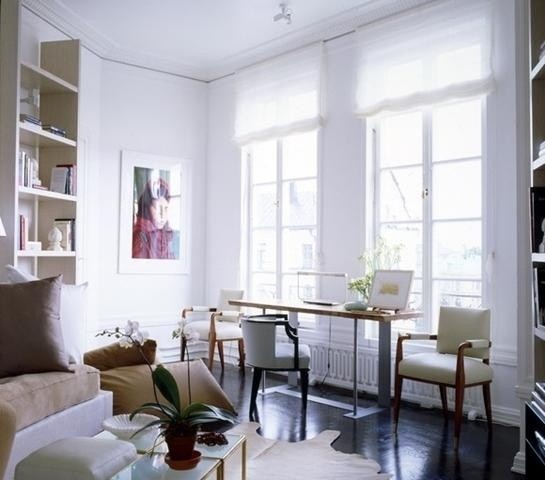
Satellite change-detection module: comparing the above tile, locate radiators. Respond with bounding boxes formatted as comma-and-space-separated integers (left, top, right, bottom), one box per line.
276, 335, 487, 420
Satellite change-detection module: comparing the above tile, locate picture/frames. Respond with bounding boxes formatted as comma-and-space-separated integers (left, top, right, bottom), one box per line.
368, 270, 413, 309
118, 150, 194, 274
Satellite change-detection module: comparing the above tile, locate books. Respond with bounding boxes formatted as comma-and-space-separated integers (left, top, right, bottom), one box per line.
49, 163, 77, 196
54, 218, 75, 251
530, 187, 545, 253
537, 140, 545, 158
533, 267, 545, 332
527, 381, 545, 424
19, 150, 48, 191
537, 38, 545, 60
20, 113, 68, 138
17, 213, 29, 251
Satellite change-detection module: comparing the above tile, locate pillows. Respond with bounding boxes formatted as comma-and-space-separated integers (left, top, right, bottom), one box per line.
0, 264, 78, 375
100, 358, 238, 417
84, 339, 157, 371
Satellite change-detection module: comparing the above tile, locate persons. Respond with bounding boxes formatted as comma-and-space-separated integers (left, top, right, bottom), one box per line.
132, 177, 177, 259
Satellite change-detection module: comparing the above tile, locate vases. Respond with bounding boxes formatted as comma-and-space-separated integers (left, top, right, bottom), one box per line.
164, 435, 202, 470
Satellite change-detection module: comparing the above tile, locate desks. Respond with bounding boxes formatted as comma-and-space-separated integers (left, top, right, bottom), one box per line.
228, 299, 422, 420
108, 429, 247, 480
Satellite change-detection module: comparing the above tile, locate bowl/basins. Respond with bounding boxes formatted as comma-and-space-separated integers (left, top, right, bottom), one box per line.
100, 413, 162, 440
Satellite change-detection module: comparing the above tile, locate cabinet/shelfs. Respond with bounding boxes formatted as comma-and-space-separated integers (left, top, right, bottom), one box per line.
529, 48, 545, 416
524, 402, 545, 479
0, 0, 82, 285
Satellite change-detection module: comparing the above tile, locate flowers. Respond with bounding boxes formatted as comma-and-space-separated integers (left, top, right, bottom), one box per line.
96, 319, 240, 458
348, 233, 407, 298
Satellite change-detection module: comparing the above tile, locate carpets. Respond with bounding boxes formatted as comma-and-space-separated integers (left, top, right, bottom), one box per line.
205, 421, 394, 480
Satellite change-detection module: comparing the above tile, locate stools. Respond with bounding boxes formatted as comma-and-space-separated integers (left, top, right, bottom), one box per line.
14, 436, 137, 480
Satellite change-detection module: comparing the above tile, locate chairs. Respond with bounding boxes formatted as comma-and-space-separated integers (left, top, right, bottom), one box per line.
180, 288, 246, 370
393, 306, 491, 449
240, 313, 311, 431
